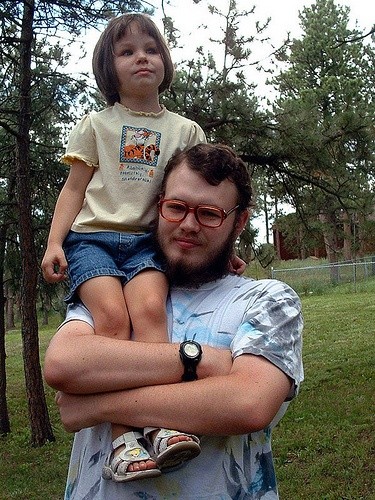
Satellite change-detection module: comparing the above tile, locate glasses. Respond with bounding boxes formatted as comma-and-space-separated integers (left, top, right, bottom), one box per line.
154, 193, 243, 228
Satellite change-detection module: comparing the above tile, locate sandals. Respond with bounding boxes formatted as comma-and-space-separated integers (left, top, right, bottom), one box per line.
101, 431, 161, 482
144, 427, 202, 469
